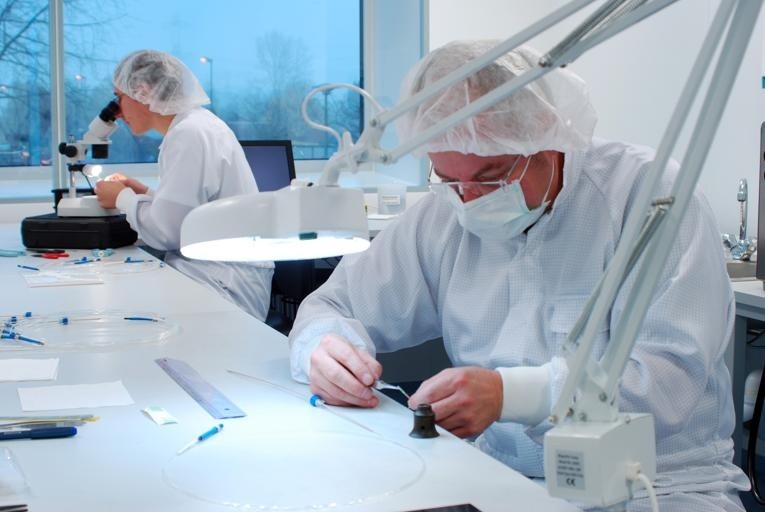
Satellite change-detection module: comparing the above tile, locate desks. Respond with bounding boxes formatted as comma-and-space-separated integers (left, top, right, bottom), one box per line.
726, 274, 765, 511
0, 218, 579, 512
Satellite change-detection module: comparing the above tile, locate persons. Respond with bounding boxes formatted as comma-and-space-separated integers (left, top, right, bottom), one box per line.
94, 50, 276, 323
287, 43, 753, 512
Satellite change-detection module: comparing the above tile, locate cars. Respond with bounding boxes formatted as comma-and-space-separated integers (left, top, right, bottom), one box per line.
0, 142, 30, 166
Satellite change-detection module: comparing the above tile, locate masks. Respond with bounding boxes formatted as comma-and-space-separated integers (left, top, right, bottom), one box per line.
443, 182, 553, 243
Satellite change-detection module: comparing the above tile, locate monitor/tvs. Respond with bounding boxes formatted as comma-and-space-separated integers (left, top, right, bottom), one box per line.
238, 139, 296, 192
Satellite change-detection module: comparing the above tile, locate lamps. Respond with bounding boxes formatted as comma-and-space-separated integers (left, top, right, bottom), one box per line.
179, 0, 763, 512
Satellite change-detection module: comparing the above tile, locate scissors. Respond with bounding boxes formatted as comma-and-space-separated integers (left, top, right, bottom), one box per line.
32, 252, 70, 259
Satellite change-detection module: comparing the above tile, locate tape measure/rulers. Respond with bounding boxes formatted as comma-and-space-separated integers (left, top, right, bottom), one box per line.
156, 359, 245, 419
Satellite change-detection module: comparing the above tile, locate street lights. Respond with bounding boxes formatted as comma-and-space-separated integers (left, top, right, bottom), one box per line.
200, 56, 213, 110
312, 83, 338, 159
75, 75, 87, 119
0, 84, 30, 124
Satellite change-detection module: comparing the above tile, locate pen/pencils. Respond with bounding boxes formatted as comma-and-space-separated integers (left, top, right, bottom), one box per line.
1, 428, 77, 440
17, 264, 39, 271
26, 249, 65, 252
177, 424, 223, 454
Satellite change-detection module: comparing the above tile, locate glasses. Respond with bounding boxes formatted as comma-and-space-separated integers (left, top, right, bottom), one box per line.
427, 160, 520, 196
